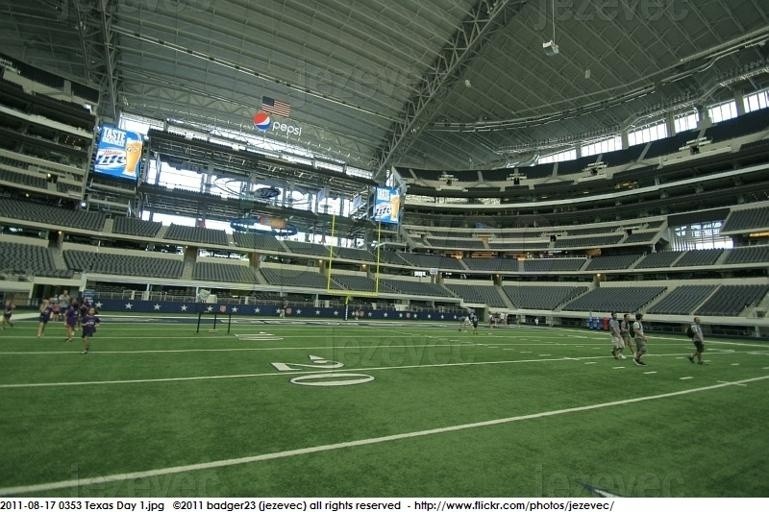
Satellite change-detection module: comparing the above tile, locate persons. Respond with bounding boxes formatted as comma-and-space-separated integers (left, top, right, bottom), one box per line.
279, 296, 289, 318
36, 289, 102, 354
0, 300, 16, 330
609, 312, 650, 366
686, 317, 705, 365
459, 310, 522, 335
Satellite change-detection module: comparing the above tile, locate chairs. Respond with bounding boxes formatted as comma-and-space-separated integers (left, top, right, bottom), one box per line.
0, 69, 768, 337
